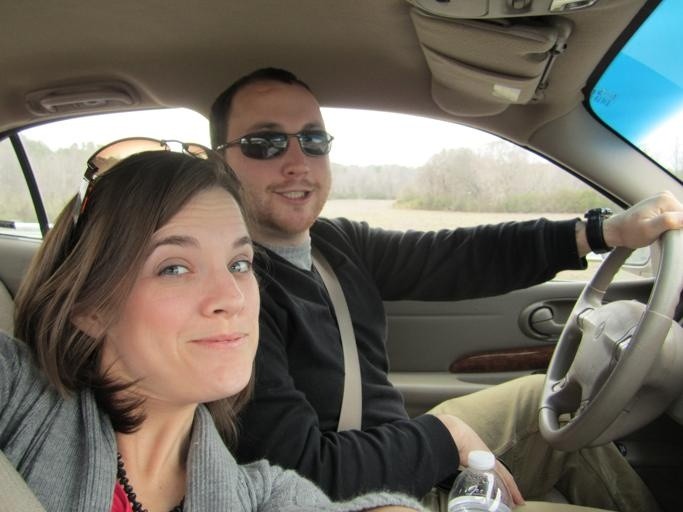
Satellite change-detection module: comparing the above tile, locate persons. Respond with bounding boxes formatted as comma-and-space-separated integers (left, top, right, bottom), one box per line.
0, 151, 426, 511
208, 63, 682, 511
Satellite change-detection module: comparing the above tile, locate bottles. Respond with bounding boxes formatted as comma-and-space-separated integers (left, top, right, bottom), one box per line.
445, 448, 514, 512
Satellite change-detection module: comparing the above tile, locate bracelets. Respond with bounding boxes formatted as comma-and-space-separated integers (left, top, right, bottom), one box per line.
583, 206, 613, 254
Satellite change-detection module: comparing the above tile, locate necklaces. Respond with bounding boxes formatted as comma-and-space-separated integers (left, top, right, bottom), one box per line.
117, 452, 185, 512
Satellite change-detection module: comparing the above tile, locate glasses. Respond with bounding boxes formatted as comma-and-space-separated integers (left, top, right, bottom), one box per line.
217, 130, 333, 159
72, 138, 236, 232
